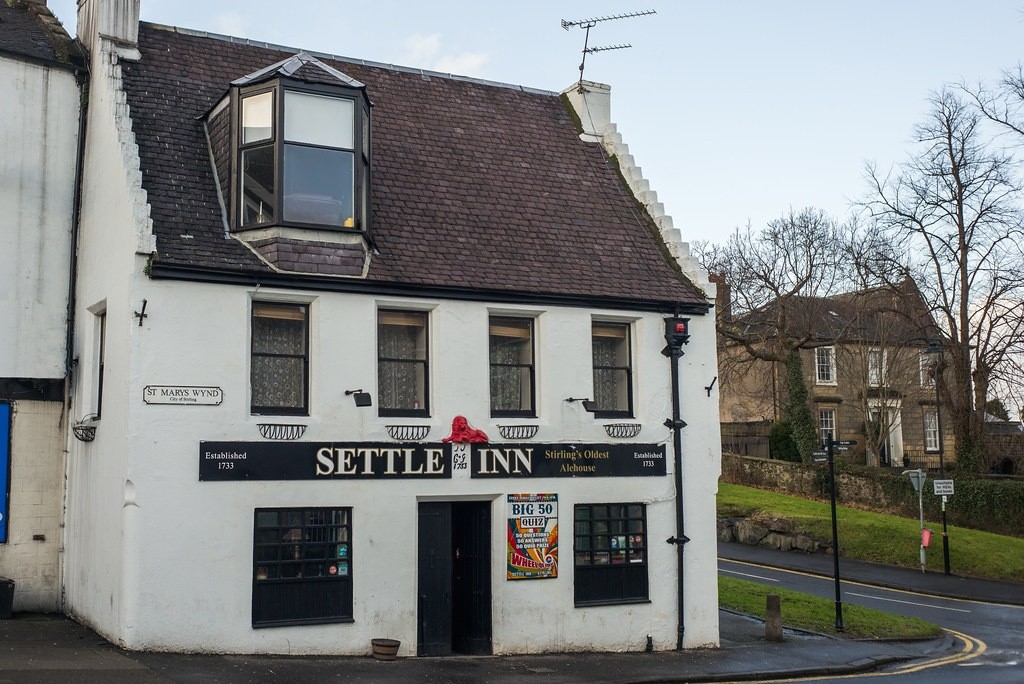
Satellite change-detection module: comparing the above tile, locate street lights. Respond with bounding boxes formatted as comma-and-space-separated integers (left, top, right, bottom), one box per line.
858, 307, 951, 574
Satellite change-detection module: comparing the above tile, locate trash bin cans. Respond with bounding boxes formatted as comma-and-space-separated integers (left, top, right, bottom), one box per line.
0, 577, 16, 619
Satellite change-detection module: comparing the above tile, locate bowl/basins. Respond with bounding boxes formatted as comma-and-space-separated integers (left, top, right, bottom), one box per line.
371, 638, 400, 660
